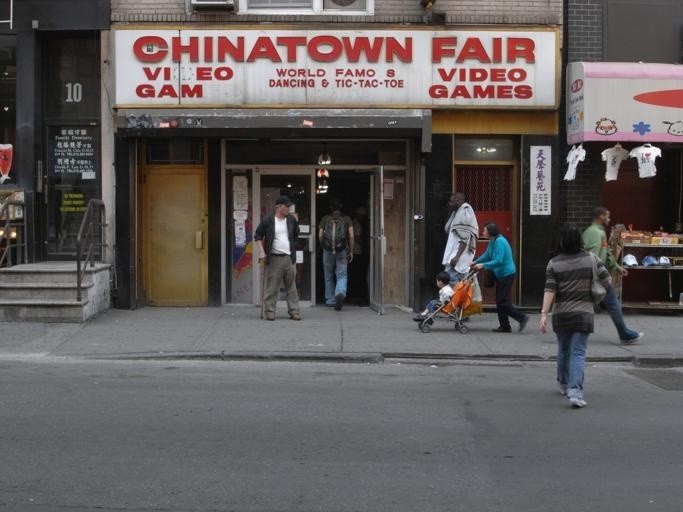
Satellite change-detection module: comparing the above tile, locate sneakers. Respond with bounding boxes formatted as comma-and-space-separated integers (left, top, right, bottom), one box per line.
493, 325, 511, 332
621, 332, 644, 345
292, 313, 300, 319
413, 314, 428, 321
335, 294, 343, 310
559, 388, 566, 394
266, 312, 274, 320
519, 316, 529, 331
570, 398, 586, 407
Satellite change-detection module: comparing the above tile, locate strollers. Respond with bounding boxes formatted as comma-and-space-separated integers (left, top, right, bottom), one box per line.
416, 265, 483, 336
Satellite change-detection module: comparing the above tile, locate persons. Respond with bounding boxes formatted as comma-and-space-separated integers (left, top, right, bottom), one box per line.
469, 222, 529, 333
538, 223, 613, 408
254, 195, 302, 321
413, 272, 455, 322
442, 192, 480, 321
579, 205, 643, 344
353, 207, 366, 254
316, 197, 355, 311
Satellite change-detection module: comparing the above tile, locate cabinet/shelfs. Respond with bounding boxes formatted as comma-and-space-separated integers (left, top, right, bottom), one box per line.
609, 223, 683, 309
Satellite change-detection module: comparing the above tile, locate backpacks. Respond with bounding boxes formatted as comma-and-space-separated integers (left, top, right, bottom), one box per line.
321, 213, 347, 254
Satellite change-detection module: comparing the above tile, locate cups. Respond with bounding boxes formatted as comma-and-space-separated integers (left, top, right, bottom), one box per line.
0, 143, 12, 179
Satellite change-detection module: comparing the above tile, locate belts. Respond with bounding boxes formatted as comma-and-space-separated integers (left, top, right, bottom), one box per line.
271, 253, 287, 256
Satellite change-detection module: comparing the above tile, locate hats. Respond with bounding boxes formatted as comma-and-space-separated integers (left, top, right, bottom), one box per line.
658, 256, 670, 265
623, 254, 638, 266
641, 256, 657, 266
276, 195, 292, 207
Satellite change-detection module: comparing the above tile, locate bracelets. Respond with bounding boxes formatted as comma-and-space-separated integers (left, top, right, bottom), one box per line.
541, 310, 548, 313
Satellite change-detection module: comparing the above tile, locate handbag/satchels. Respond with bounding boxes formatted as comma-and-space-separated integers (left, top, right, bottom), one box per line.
591, 278, 606, 305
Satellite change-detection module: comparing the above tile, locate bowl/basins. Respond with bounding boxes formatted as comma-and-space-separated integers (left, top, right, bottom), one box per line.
654, 232, 668, 237
